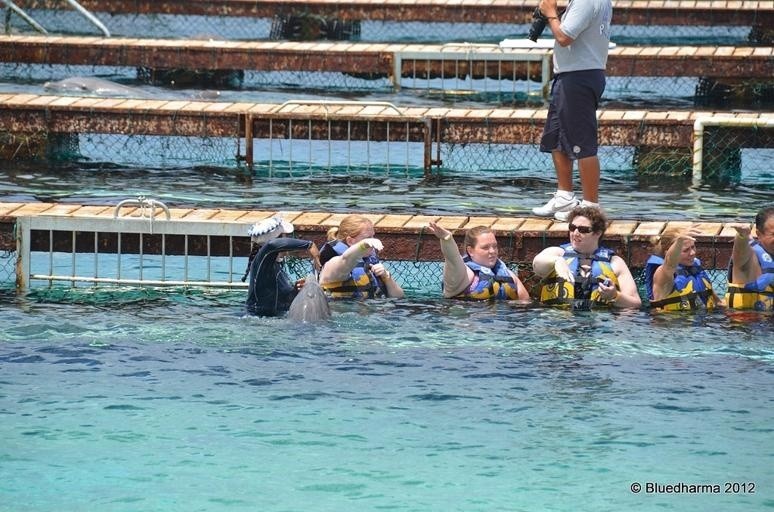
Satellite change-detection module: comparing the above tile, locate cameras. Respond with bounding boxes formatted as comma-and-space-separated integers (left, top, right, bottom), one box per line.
527, 5, 550, 42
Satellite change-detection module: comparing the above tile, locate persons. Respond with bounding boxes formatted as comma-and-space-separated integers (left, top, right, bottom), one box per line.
424, 222, 530, 301
725, 204, 773, 311
644, 223, 722, 310
313, 214, 404, 300
532, 205, 642, 308
529, 0, 613, 221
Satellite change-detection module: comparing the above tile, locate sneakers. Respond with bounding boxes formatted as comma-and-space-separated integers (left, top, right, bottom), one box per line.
532, 191, 581, 216
551, 203, 601, 221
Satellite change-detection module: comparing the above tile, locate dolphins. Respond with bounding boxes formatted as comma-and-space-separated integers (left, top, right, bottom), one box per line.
44, 76, 221, 99
288, 271, 332, 323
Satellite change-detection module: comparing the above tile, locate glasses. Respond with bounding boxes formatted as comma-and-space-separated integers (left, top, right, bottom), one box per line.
568, 223, 599, 233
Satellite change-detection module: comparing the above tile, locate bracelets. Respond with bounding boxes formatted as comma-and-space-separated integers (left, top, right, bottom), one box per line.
735, 232, 745, 240
360, 239, 368, 251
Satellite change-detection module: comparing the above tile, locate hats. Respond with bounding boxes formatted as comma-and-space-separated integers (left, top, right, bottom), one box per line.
248, 217, 295, 245
